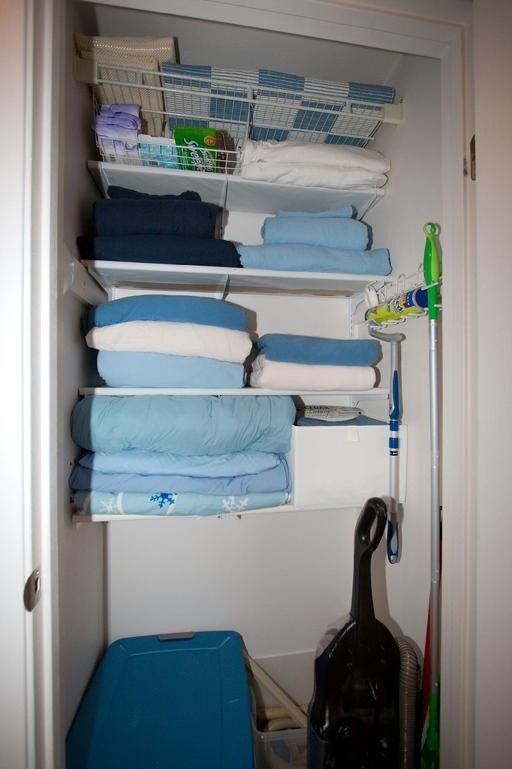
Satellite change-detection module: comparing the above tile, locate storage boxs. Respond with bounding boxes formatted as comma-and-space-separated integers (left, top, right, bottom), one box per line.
286, 424, 407, 508
251, 712, 307, 769
66, 631, 252, 768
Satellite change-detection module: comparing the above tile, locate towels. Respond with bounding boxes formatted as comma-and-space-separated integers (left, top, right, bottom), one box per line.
106, 185, 202, 201
276, 205, 352, 218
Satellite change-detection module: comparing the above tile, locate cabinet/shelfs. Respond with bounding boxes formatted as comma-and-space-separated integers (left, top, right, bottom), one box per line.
31, 0, 474, 769
